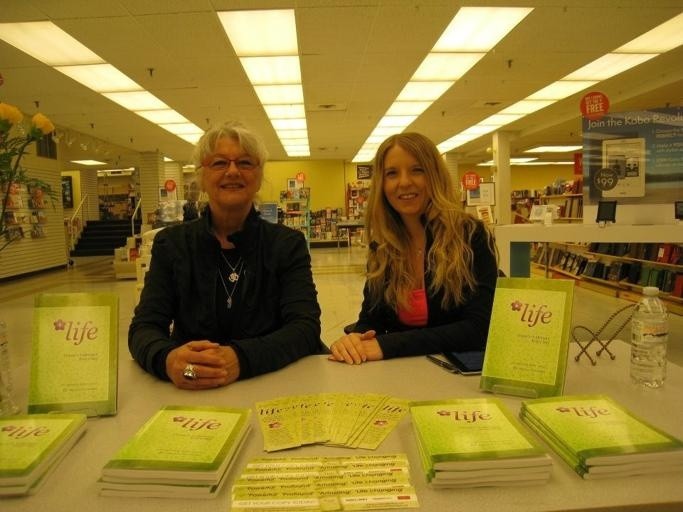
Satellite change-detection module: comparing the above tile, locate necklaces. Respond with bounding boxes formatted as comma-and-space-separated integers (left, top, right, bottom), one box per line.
410, 244, 427, 257
219, 251, 244, 282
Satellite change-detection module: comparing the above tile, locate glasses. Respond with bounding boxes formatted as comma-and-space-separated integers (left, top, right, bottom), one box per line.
207, 154, 260, 170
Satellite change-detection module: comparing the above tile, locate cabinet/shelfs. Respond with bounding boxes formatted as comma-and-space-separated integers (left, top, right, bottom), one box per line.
582, 243, 683, 317
509, 193, 584, 280
280, 187, 311, 256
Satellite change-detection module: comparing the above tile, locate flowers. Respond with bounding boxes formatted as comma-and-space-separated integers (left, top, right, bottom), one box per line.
0, 104, 55, 257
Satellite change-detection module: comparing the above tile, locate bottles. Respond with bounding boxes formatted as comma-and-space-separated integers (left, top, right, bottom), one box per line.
629, 285, 669, 389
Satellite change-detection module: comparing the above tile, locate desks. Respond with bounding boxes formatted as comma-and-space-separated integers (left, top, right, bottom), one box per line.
1, 338, 682, 510
335, 222, 368, 247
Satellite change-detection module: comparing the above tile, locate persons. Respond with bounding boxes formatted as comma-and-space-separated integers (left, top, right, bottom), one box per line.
183, 194, 199, 222
326, 133, 500, 366
128, 119, 321, 388
511, 204, 518, 224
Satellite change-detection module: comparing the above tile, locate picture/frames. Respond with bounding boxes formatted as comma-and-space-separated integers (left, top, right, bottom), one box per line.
61, 175, 74, 209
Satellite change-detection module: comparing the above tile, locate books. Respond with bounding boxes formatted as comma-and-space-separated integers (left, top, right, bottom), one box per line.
479, 278, 575, 397
531, 242, 682, 297
407, 398, 554, 488
519, 394, 682, 480
2, 290, 253, 497
